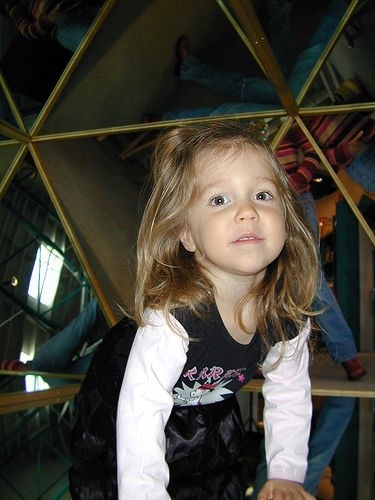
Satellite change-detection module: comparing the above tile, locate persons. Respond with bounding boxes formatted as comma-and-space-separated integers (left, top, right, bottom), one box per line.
276, 121, 367, 381
73, 121, 323, 500
0, 0, 375, 500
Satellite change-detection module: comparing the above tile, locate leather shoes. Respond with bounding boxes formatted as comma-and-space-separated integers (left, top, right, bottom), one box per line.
4, 359, 31, 372
342, 358, 367, 379
